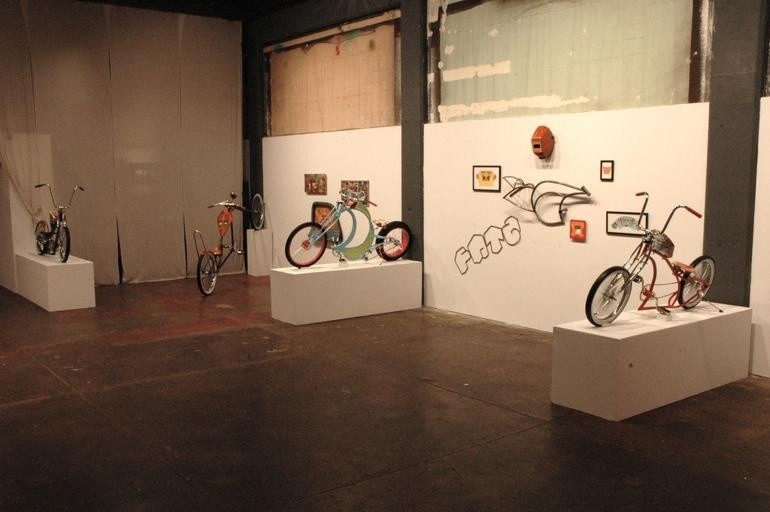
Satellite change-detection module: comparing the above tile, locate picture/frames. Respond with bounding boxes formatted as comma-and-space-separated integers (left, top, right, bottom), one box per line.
570, 220, 585, 242
606, 211, 648, 237
311, 201, 344, 247
472, 164, 501, 193
600, 160, 614, 182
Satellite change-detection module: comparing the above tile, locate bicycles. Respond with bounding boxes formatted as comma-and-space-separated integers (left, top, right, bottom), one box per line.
284, 187, 413, 268
34, 183, 86, 264
194, 189, 267, 299
583, 191, 730, 328
